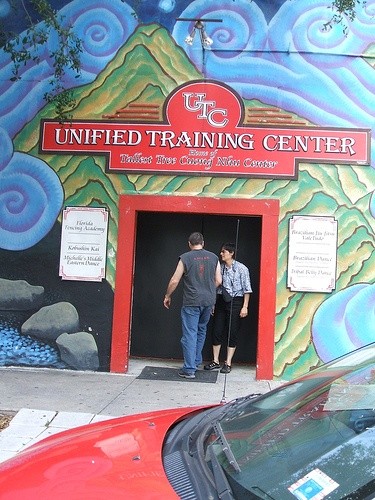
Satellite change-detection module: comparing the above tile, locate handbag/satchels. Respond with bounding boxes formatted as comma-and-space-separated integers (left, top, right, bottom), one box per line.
221, 265, 242, 303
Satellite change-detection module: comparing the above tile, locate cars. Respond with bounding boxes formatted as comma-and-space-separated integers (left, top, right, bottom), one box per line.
0, 342, 375, 500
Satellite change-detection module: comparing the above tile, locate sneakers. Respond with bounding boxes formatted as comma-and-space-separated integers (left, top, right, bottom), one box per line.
220, 364, 231, 373
177, 369, 195, 378
204, 361, 221, 370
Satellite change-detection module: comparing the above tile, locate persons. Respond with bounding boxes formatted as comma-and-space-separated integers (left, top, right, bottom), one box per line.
204, 243, 252, 373
163, 232, 222, 378
